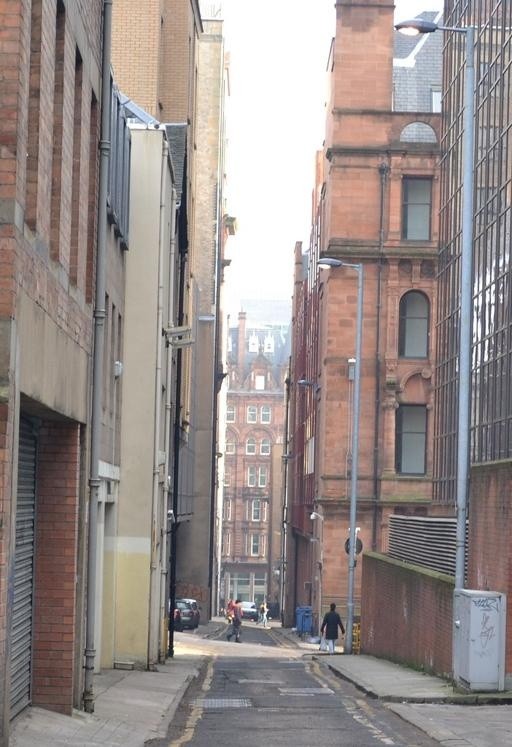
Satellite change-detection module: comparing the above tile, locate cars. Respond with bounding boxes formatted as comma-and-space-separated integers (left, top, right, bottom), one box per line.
241, 602, 258, 622
173, 599, 202, 632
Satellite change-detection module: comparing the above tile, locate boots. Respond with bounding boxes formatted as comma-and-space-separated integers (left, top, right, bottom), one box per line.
236, 637, 242, 644
226, 635, 233, 642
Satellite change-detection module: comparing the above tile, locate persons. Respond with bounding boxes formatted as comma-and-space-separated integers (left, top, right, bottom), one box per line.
320, 602, 345, 656
227, 598, 243, 643
256, 602, 270, 627
226, 600, 234, 623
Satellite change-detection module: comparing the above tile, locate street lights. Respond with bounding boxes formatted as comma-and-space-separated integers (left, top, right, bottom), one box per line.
318, 258, 364, 655
396, 20, 475, 588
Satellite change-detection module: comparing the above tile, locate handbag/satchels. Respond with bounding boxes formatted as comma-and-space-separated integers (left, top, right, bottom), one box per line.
318, 631, 328, 652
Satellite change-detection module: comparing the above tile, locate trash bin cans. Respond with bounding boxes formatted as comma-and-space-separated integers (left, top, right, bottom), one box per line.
296, 605, 313, 633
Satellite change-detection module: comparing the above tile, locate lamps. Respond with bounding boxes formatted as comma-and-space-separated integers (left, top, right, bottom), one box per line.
310, 512, 324, 520
